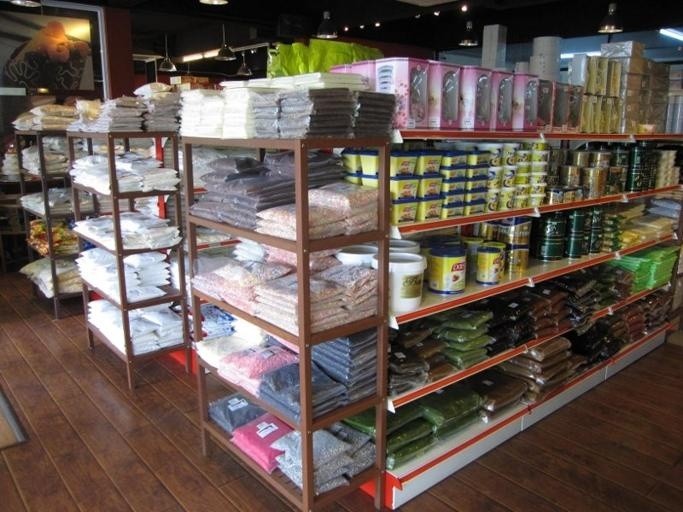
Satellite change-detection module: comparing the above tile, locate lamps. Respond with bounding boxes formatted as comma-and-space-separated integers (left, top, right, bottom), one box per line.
594, 2, 626, 37
315, 0, 340, 41
159, 32, 177, 73
213, 23, 254, 75
458, 15, 480, 47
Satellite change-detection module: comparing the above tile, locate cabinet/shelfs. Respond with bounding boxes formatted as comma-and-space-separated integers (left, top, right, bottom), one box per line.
187, 133, 683, 510
11, 133, 152, 320
67, 131, 230, 395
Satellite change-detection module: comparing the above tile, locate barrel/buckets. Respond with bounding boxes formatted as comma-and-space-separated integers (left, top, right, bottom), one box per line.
336, 239, 429, 314
